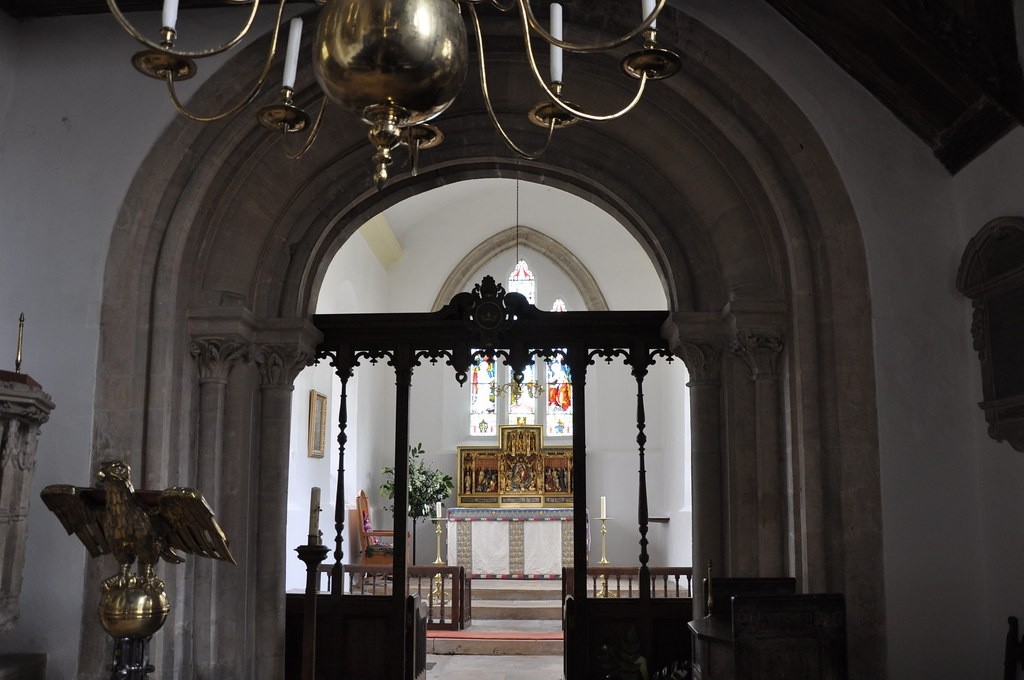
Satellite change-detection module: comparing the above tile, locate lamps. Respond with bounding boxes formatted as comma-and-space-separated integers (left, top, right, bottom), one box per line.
108, 1, 683, 200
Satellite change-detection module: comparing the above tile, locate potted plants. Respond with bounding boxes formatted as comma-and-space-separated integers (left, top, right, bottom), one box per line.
379, 442, 455, 570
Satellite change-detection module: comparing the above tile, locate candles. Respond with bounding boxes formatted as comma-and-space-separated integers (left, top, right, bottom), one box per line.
436, 501, 443, 518
308, 486, 321, 536
601, 496, 607, 520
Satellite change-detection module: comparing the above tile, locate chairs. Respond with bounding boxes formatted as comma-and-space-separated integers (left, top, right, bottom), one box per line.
356, 490, 412, 591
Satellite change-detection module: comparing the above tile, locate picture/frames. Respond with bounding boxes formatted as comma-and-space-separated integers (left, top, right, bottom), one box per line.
309, 389, 328, 459
